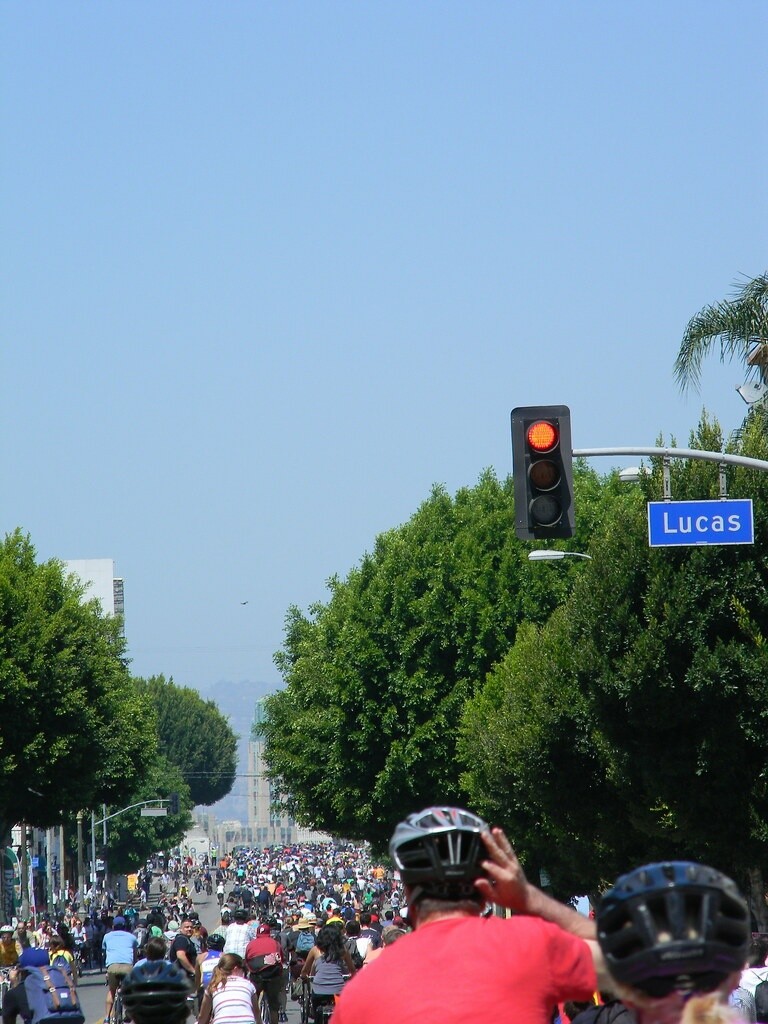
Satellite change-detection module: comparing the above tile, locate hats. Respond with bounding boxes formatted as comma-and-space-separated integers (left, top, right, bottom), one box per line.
292, 918, 314, 929
113, 916, 125, 928
256, 924, 271, 938
344, 902, 352, 905
18, 947, 50, 972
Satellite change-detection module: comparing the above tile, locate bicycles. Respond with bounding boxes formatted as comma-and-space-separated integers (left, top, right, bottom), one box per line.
0, 966, 20, 1011
213, 892, 412, 1024
71, 944, 87, 980
111, 972, 128, 1024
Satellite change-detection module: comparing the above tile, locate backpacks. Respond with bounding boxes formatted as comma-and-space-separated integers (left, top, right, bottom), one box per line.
23, 965, 85, 1024
295, 930, 315, 953
750, 969, 768, 1021
52, 950, 71, 975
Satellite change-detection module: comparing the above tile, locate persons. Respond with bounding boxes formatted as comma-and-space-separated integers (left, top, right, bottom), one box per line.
596, 861, 751, 1024
739, 933, 768, 1024
728, 986, 756, 1024
327, 806, 611, 1024
0, 842, 409, 1024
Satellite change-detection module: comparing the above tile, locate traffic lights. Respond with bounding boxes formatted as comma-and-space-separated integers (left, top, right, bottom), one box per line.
525, 419, 571, 537
96, 858, 104, 872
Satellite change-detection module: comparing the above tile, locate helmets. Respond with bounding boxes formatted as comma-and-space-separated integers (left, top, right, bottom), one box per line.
206, 934, 225, 947
0, 925, 14, 935
596, 861, 751, 986
388, 807, 495, 884
121, 961, 190, 1015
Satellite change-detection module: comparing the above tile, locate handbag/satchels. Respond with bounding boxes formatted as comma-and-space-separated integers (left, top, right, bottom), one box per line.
291, 977, 305, 1001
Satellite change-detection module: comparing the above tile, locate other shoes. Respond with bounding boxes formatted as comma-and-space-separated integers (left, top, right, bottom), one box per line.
103, 1018, 110, 1024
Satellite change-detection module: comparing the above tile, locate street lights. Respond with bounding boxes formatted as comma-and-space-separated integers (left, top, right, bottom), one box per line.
187, 839, 205, 856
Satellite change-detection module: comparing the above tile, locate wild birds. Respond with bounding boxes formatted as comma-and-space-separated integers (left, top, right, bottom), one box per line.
240, 600, 249, 606
